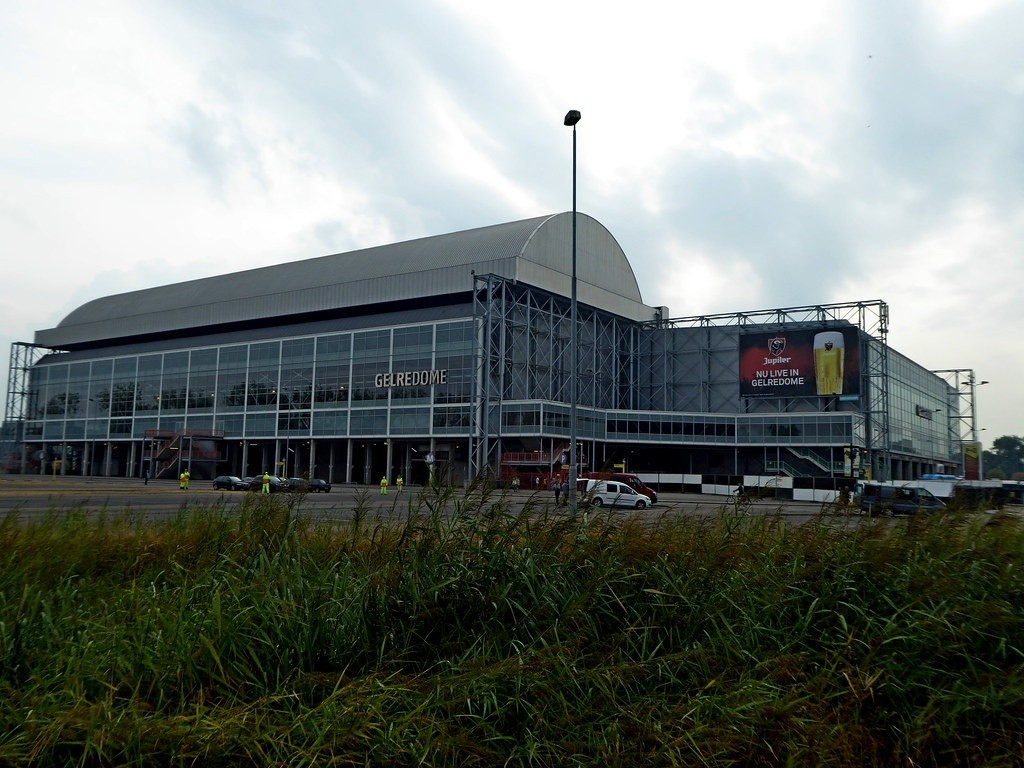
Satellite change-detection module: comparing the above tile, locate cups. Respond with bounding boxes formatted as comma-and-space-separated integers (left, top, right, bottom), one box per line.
813, 331, 845, 396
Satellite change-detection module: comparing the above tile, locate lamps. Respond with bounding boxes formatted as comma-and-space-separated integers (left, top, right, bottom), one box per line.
920, 408, 941, 412
977, 428, 986, 431
976, 380, 989, 385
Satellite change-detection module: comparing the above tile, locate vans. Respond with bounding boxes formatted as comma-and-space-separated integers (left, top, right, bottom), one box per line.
590, 472, 658, 503
852, 482, 947, 519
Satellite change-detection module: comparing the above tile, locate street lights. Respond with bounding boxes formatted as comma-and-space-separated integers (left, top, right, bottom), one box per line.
563, 109, 584, 517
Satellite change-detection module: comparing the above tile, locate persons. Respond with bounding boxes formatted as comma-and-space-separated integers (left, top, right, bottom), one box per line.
733, 482, 745, 497
380, 475, 389, 495
179, 468, 191, 490
839, 482, 852, 512
261, 472, 270, 495
144, 469, 150, 485
512, 476, 521, 493
396, 474, 404, 493
534, 475, 569, 505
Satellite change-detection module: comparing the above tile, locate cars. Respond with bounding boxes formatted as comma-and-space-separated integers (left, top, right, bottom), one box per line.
246, 476, 332, 493
212, 476, 250, 491
577, 479, 651, 510
